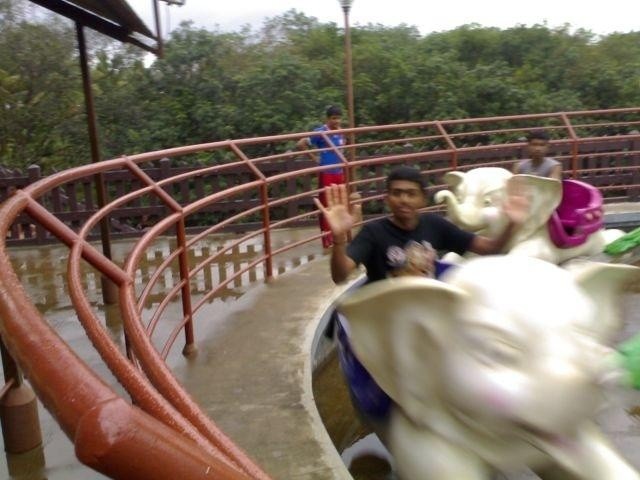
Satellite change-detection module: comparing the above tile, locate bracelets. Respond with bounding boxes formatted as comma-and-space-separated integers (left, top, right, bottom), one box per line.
331, 239, 352, 247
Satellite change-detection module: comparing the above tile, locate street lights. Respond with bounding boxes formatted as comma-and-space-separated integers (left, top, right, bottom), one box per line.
340, 0, 359, 193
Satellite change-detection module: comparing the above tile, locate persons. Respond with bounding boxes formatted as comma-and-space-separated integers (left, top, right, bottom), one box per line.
314, 166, 534, 286
298, 107, 348, 255
514, 132, 562, 185
